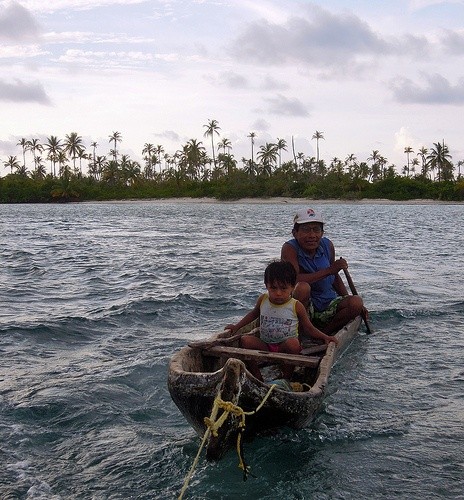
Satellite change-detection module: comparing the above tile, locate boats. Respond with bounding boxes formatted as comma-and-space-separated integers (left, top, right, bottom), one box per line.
169, 309, 366, 462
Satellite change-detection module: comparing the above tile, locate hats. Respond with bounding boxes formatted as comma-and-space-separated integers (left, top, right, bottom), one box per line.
293, 207, 325, 223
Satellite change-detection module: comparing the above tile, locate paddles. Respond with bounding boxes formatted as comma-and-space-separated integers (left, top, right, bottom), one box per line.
187, 323, 261, 351
340, 255, 374, 335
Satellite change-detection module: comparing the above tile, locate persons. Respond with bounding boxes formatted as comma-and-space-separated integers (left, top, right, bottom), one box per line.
224, 261, 339, 385
278, 207, 368, 337
